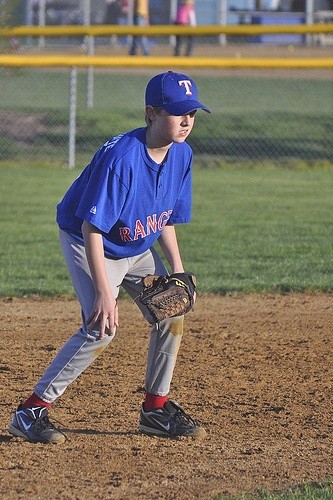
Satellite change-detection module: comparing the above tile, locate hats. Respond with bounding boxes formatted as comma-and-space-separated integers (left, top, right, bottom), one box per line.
146, 70, 212, 116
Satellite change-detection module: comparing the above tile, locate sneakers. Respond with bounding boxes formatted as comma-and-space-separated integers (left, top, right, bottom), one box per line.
9, 405, 65, 445
140, 400, 205, 438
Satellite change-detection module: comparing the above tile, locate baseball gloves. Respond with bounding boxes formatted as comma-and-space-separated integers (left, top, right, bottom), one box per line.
132, 272, 196, 322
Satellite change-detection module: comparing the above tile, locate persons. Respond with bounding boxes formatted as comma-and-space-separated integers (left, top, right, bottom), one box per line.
128, 0, 152, 57
170, 1, 197, 57
6, 71, 221, 453
